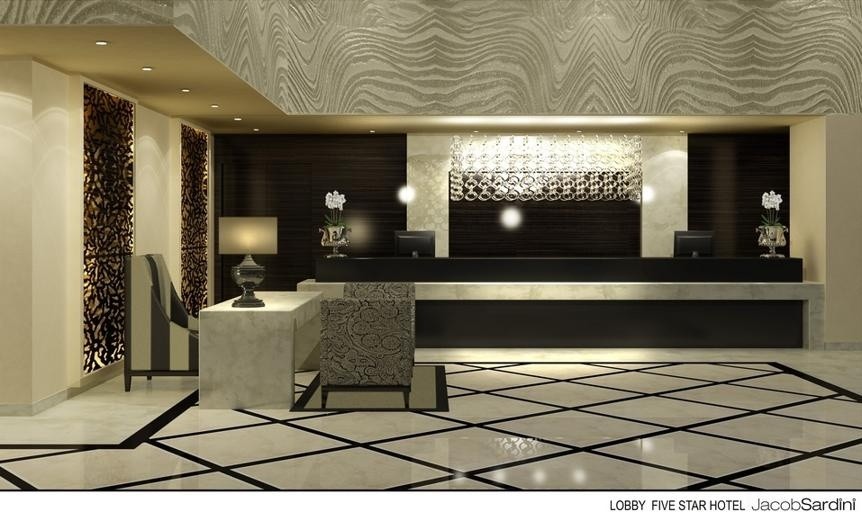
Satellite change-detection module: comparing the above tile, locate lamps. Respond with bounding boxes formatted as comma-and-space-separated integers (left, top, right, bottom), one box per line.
215, 215, 277, 309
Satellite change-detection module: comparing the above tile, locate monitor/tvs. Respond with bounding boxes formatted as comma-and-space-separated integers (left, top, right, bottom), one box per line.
674, 230, 714, 258
395, 231, 436, 258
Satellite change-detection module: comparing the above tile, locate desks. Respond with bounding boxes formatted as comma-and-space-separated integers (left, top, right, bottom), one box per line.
197, 290, 322, 412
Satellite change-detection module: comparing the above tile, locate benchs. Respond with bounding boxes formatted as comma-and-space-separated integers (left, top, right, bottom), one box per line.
318, 282, 417, 408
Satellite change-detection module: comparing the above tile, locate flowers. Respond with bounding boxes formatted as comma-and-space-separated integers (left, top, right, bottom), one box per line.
321, 190, 349, 228
758, 191, 787, 230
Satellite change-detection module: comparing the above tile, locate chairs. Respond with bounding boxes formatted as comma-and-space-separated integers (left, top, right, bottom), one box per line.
122, 253, 201, 392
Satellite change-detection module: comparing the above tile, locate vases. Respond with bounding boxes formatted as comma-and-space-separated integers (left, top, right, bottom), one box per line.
318, 226, 352, 259
757, 225, 789, 259
